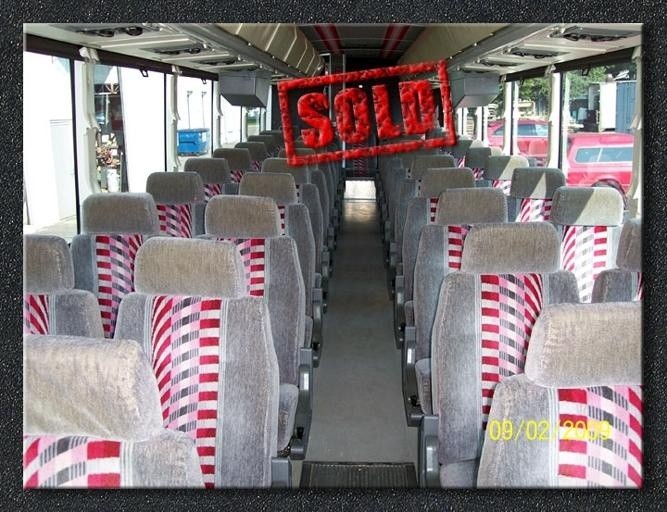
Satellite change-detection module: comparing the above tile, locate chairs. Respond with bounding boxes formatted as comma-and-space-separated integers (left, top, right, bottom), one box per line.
22, 128, 345, 489
375, 123, 643, 489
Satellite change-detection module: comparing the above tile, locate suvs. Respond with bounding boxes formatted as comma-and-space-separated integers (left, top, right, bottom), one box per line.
564, 130, 634, 209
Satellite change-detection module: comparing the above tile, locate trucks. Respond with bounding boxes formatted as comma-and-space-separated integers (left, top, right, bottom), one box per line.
486, 78, 636, 134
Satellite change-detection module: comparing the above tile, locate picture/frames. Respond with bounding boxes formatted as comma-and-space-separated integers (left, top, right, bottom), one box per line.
1, 0, 667, 512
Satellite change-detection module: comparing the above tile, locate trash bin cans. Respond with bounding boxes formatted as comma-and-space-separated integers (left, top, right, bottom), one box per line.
176, 128, 210, 156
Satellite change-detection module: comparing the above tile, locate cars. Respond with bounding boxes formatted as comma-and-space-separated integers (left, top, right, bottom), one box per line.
246, 106, 265, 119
485, 113, 573, 161
